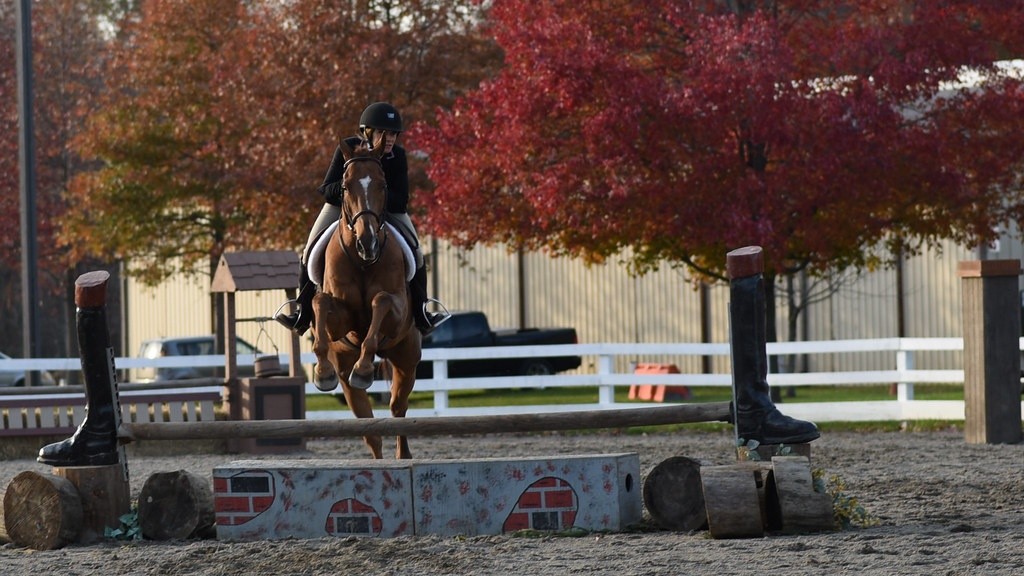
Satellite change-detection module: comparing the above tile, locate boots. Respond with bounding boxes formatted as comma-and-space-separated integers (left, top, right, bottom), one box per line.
36, 302, 123, 467
729, 275, 821, 445
276, 254, 315, 336
409, 254, 445, 337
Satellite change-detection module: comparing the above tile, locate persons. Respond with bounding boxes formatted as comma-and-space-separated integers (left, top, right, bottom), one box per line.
275, 102, 444, 336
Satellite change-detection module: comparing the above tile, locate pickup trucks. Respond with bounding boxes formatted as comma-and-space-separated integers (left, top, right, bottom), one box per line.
331, 311, 581, 408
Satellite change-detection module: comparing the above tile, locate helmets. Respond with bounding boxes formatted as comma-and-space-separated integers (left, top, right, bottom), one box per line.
359, 102, 401, 134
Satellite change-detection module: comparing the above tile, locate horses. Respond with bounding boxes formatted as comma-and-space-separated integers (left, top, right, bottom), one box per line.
309, 129, 421, 459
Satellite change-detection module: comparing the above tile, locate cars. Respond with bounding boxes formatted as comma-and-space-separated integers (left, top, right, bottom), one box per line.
133, 336, 265, 382
1, 351, 56, 387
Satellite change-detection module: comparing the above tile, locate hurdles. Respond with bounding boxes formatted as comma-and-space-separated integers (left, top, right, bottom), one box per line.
101, 298, 742, 483
